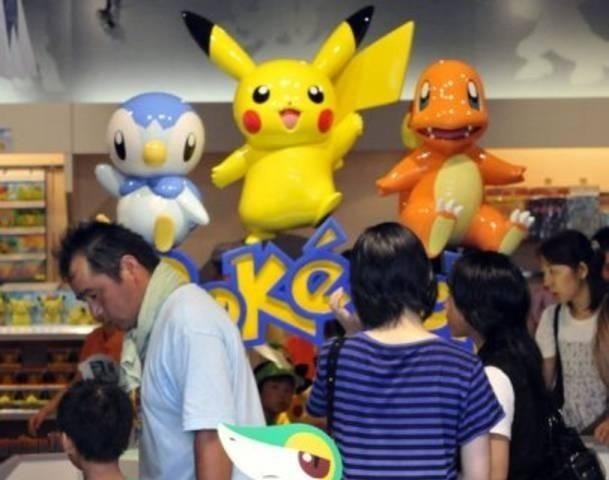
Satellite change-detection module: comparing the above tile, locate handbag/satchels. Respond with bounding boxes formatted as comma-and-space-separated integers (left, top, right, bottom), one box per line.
530, 404, 604, 479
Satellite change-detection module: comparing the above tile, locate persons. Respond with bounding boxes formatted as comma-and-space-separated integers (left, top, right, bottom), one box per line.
444, 247, 547, 480
54, 376, 137, 479
595, 225, 609, 288
303, 220, 506, 479
534, 229, 609, 479
252, 360, 296, 426
50, 217, 269, 479
26, 316, 127, 440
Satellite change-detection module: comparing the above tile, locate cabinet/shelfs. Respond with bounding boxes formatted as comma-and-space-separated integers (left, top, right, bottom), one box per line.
0, 152, 104, 422
